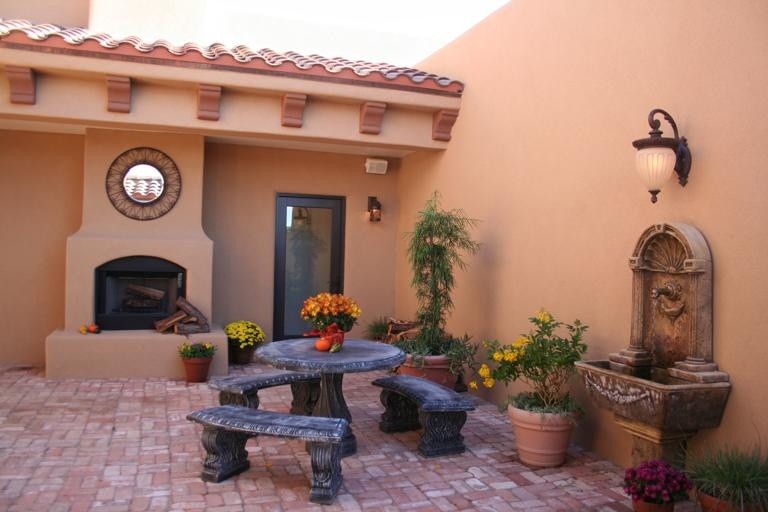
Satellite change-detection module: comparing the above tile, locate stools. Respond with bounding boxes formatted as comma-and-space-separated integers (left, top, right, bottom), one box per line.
186, 404, 349, 505
207, 370, 321, 438
371, 375, 475, 456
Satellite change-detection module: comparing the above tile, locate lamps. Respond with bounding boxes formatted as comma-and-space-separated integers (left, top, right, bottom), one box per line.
632, 108, 691, 203
294, 207, 306, 226
369, 202, 382, 224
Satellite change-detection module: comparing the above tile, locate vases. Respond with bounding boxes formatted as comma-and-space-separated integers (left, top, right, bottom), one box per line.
320, 325, 344, 347
183, 358, 211, 382
234, 346, 250, 364
632, 499, 674, 512
508, 403, 580, 468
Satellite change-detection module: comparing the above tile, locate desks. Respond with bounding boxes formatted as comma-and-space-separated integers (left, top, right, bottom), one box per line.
253, 338, 406, 459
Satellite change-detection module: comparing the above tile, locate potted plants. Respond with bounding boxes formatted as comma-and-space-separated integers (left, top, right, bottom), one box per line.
365, 315, 395, 346
667, 441, 768, 511
393, 191, 486, 391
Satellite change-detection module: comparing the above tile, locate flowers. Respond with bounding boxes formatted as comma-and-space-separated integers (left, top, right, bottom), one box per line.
224, 320, 266, 351
300, 292, 362, 331
623, 460, 691, 502
176, 338, 218, 359
469, 308, 590, 425
